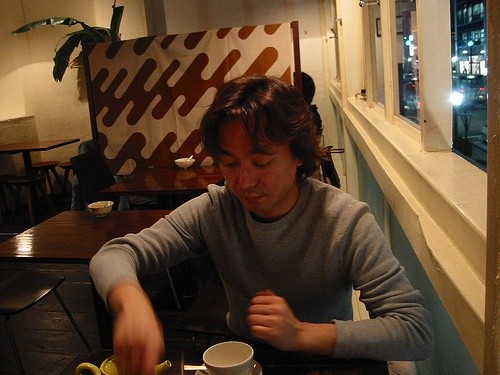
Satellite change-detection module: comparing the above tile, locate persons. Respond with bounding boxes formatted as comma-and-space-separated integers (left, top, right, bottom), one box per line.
292, 72, 322, 134
89, 74, 434, 375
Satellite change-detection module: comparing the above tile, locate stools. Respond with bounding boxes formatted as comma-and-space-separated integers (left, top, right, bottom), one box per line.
0, 272, 91, 375
0, 160, 75, 233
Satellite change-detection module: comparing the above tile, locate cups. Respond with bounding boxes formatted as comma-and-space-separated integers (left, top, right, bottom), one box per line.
202, 341, 254, 375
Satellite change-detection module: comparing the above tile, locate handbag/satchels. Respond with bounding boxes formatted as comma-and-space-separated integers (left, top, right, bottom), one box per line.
320, 145, 345, 189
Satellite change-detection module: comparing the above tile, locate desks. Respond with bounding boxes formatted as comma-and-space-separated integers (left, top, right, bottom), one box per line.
0, 138, 80, 228
0, 211, 173, 352
102, 167, 226, 211
58, 317, 388, 375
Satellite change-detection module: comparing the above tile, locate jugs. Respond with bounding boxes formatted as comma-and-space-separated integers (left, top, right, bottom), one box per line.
74, 352, 172, 375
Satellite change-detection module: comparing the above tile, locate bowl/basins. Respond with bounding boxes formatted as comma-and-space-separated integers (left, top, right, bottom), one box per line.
174, 158, 195, 168
87, 200, 114, 217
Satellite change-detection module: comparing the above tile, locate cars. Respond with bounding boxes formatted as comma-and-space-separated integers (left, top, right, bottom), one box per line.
399, 72, 486, 112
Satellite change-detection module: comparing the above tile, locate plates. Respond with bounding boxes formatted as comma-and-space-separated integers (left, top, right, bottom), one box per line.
194, 362, 262, 375
